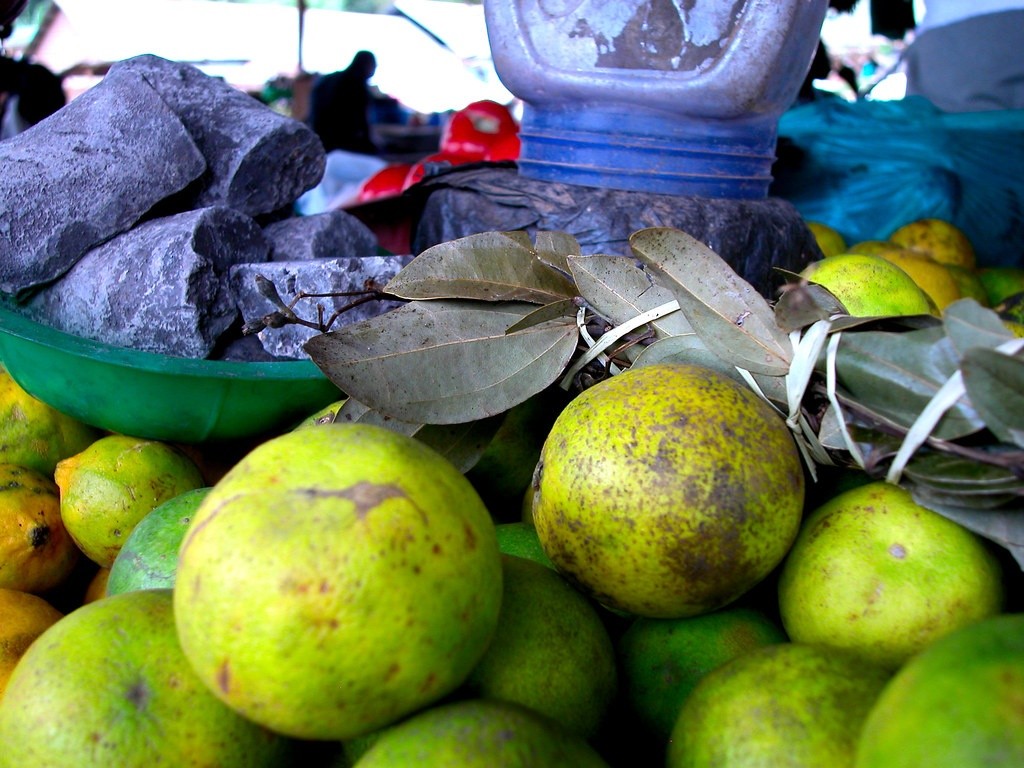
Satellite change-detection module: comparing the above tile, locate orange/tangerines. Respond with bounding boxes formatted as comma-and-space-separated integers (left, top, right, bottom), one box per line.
2, 227, 1024, 768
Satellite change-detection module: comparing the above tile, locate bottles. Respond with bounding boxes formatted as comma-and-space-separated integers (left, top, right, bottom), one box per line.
475, 0, 834, 195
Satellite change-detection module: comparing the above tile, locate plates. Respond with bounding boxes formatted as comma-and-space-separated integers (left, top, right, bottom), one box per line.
0, 288, 380, 438
